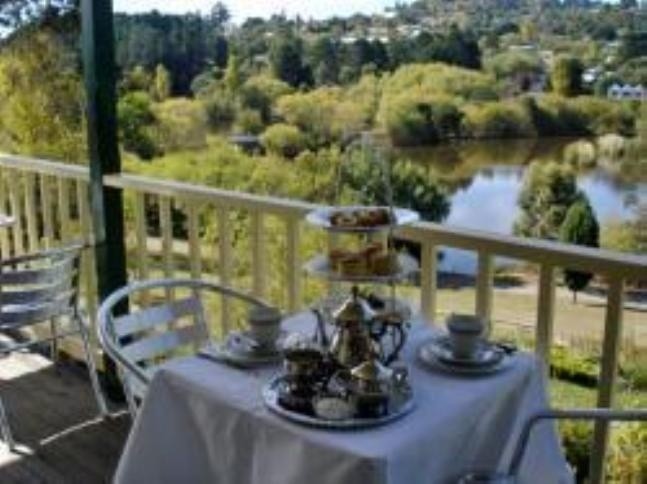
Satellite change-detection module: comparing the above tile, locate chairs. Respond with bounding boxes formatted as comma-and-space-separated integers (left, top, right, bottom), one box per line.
453, 409, 647, 484
0, 244, 112, 451
95, 279, 287, 420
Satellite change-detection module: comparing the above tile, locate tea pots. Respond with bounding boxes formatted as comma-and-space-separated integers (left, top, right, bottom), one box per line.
310, 285, 407, 374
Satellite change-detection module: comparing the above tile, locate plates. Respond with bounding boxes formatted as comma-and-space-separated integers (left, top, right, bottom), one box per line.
223, 325, 291, 363
415, 332, 519, 376
259, 369, 419, 429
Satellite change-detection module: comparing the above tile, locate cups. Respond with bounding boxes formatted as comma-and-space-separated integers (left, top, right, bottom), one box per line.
444, 311, 494, 359
236, 303, 282, 348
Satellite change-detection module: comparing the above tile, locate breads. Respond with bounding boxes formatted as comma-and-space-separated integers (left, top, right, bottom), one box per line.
326, 208, 398, 276
